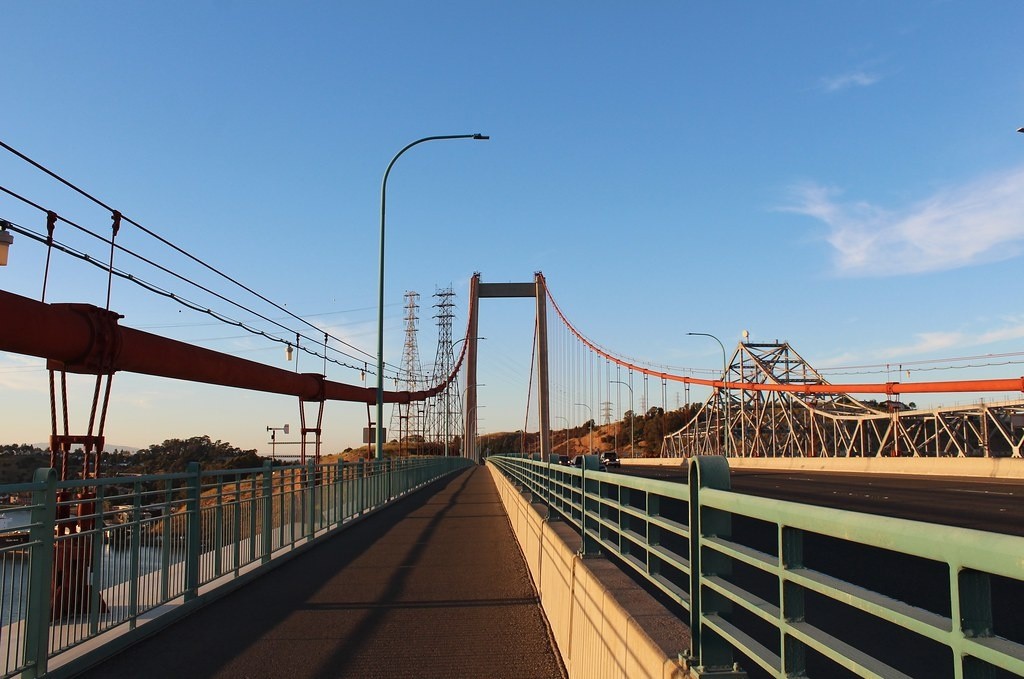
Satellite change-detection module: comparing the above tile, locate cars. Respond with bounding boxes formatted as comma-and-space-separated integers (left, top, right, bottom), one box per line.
571, 456, 607, 472
559, 455, 571, 466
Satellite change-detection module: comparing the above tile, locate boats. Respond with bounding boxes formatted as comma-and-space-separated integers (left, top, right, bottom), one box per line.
0, 530, 33, 560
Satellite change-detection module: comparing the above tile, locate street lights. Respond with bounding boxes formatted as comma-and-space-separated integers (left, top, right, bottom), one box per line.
374, 132, 491, 462
470, 418, 484, 456
460, 384, 486, 457
609, 380, 634, 458
687, 332, 729, 457
573, 403, 592, 456
555, 416, 569, 457
444, 336, 486, 458
466, 405, 485, 457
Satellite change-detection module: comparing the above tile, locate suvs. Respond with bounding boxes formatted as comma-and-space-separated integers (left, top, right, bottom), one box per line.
600, 452, 621, 469
